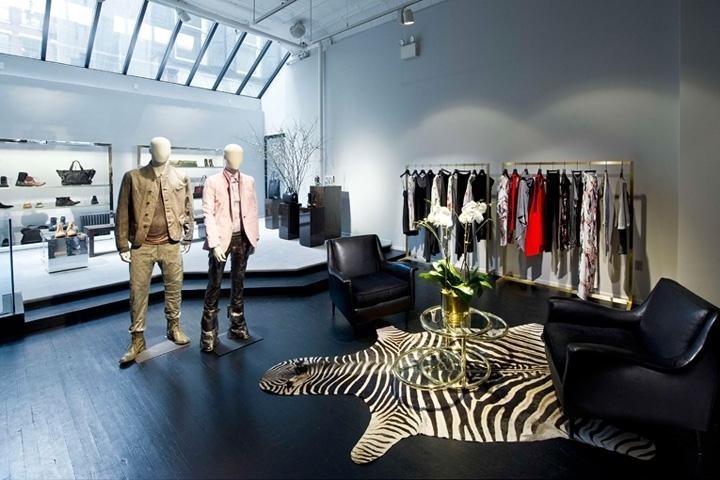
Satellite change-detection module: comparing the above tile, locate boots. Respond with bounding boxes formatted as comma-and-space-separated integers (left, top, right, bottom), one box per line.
49, 216, 78, 238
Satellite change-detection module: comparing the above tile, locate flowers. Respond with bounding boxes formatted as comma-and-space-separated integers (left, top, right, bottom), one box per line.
411, 194, 503, 299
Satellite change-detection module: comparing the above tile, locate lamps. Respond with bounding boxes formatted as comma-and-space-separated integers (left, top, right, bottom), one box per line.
398, 6, 416, 27
289, 18, 306, 35
176, 8, 191, 24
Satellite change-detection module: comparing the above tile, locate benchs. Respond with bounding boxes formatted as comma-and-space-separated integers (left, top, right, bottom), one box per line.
80, 222, 116, 255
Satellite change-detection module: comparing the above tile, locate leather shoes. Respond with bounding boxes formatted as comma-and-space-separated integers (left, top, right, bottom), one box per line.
91, 196, 99, 204
2, 238, 9, 246
209, 159, 214, 167
1, 172, 45, 187
0, 203, 13, 208
55, 197, 81, 206
205, 159, 208, 167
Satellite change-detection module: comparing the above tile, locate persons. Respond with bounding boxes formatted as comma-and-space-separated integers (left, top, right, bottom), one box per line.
114, 137, 194, 363
200, 144, 260, 352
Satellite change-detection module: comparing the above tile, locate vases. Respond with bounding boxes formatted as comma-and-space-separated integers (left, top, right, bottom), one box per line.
442, 286, 469, 318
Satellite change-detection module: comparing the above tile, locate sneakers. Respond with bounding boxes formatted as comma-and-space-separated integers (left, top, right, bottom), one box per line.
23, 202, 31, 208
35, 202, 43, 208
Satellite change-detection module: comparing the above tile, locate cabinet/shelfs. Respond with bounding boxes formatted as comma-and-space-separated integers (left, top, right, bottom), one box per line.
134, 145, 230, 214
1, 137, 116, 242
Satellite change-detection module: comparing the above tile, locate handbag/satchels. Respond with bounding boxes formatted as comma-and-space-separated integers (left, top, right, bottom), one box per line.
56, 160, 96, 185
195, 175, 207, 193
20, 228, 42, 244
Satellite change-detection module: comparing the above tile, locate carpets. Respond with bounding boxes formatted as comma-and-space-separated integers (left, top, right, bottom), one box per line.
260, 324, 658, 471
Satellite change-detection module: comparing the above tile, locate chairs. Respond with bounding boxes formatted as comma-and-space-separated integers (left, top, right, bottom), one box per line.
543, 275, 719, 457
321, 234, 415, 342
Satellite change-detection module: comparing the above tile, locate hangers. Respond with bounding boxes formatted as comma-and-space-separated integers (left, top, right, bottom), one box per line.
500, 160, 628, 186
399, 163, 495, 187
